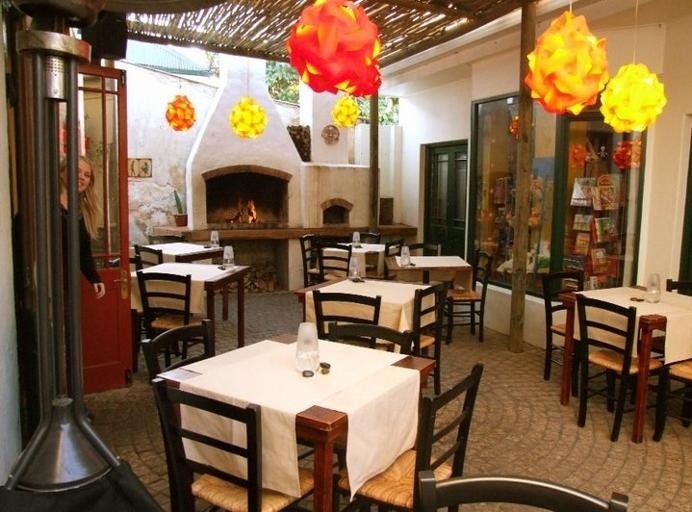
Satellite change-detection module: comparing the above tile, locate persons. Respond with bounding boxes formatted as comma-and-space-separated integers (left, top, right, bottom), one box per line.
15, 155, 107, 424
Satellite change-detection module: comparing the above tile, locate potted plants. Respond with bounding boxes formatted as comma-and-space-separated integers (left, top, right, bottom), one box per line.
173, 189, 188, 228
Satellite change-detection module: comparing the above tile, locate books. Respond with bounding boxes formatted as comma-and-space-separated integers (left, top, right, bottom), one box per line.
570, 173, 622, 291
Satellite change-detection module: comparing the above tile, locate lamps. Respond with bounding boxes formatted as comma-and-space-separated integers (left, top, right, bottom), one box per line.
520, 1, 612, 117
330, 93, 361, 128
163, 57, 198, 133
341, 60, 381, 102
596, 2, 669, 136
613, 137, 642, 170
285, 1, 385, 96
227, 69, 271, 140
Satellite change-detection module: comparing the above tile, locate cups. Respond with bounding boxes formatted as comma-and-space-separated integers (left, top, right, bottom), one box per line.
648, 273, 661, 303
352, 232, 361, 247
348, 256, 360, 279
295, 322, 320, 374
209, 230, 219, 248
222, 245, 235, 270
401, 246, 410, 266
